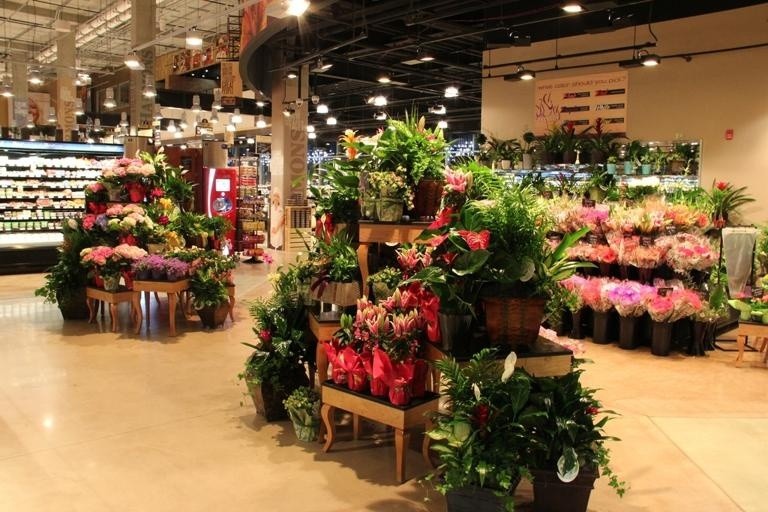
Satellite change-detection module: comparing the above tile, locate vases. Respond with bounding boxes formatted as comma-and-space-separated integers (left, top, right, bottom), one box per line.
194, 300, 229, 329
57, 284, 99, 320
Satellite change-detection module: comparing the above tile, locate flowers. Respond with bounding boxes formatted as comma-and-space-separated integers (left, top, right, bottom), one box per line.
32, 145, 234, 314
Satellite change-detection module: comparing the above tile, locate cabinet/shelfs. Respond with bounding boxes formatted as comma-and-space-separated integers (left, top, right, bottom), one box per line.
1, 140, 123, 248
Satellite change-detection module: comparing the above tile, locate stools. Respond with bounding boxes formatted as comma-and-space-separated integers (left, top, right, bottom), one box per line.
222, 281, 235, 323
86, 285, 139, 333
133, 278, 193, 336
